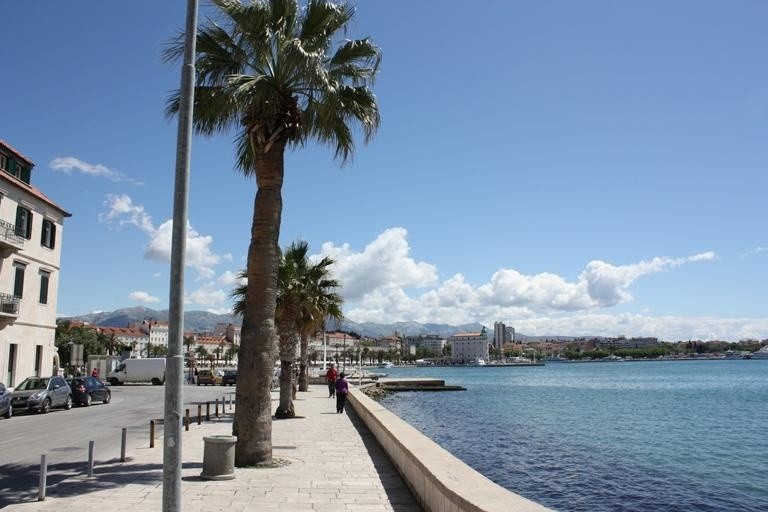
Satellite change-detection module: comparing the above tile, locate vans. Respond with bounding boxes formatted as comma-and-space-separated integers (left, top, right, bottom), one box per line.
106, 357, 166, 385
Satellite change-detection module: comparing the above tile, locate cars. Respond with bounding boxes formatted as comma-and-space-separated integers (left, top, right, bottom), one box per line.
64, 377, 111, 406
0, 381, 14, 419
10, 375, 74, 414
196, 368, 237, 386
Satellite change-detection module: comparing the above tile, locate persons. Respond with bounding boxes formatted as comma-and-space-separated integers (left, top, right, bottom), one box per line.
325, 364, 338, 399
334, 372, 349, 414
75, 363, 98, 379
